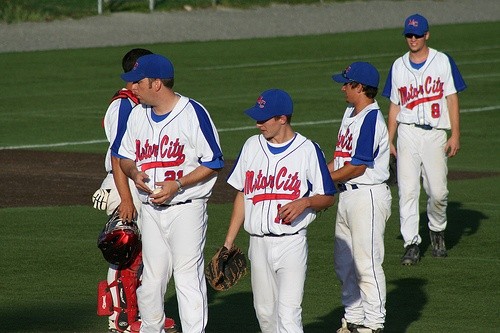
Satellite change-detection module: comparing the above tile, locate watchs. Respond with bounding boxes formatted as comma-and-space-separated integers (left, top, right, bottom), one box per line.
176, 179, 183, 192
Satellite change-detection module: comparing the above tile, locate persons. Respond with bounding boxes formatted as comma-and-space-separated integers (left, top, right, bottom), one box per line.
383, 14, 467, 264
119, 56, 223, 333
206, 89, 338, 333
101, 48, 176, 333
326, 62, 392, 333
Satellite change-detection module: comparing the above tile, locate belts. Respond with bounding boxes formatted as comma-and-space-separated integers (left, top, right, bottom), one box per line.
339, 184, 359, 191
407, 122, 432, 129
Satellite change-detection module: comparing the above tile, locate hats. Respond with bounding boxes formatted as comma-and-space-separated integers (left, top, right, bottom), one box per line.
332, 62, 380, 89
120, 53, 175, 83
245, 89, 294, 122
404, 13, 429, 36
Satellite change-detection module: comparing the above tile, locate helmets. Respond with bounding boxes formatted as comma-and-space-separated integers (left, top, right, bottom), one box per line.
98, 206, 142, 271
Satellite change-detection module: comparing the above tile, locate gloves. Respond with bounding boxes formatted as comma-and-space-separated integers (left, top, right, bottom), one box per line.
92, 190, 108, 209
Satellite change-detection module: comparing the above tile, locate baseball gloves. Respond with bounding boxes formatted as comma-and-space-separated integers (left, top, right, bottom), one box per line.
205, 245, 248, 293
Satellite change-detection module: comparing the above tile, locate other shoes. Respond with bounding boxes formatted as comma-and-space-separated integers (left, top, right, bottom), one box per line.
336, 322, 388, 333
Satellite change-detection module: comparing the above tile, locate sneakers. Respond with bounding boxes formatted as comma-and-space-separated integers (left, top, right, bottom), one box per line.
400, 236, 423, 266
430, 231, 447, 257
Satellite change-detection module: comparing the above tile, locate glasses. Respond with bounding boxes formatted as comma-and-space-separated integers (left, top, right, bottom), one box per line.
405, 33, 426, 39
